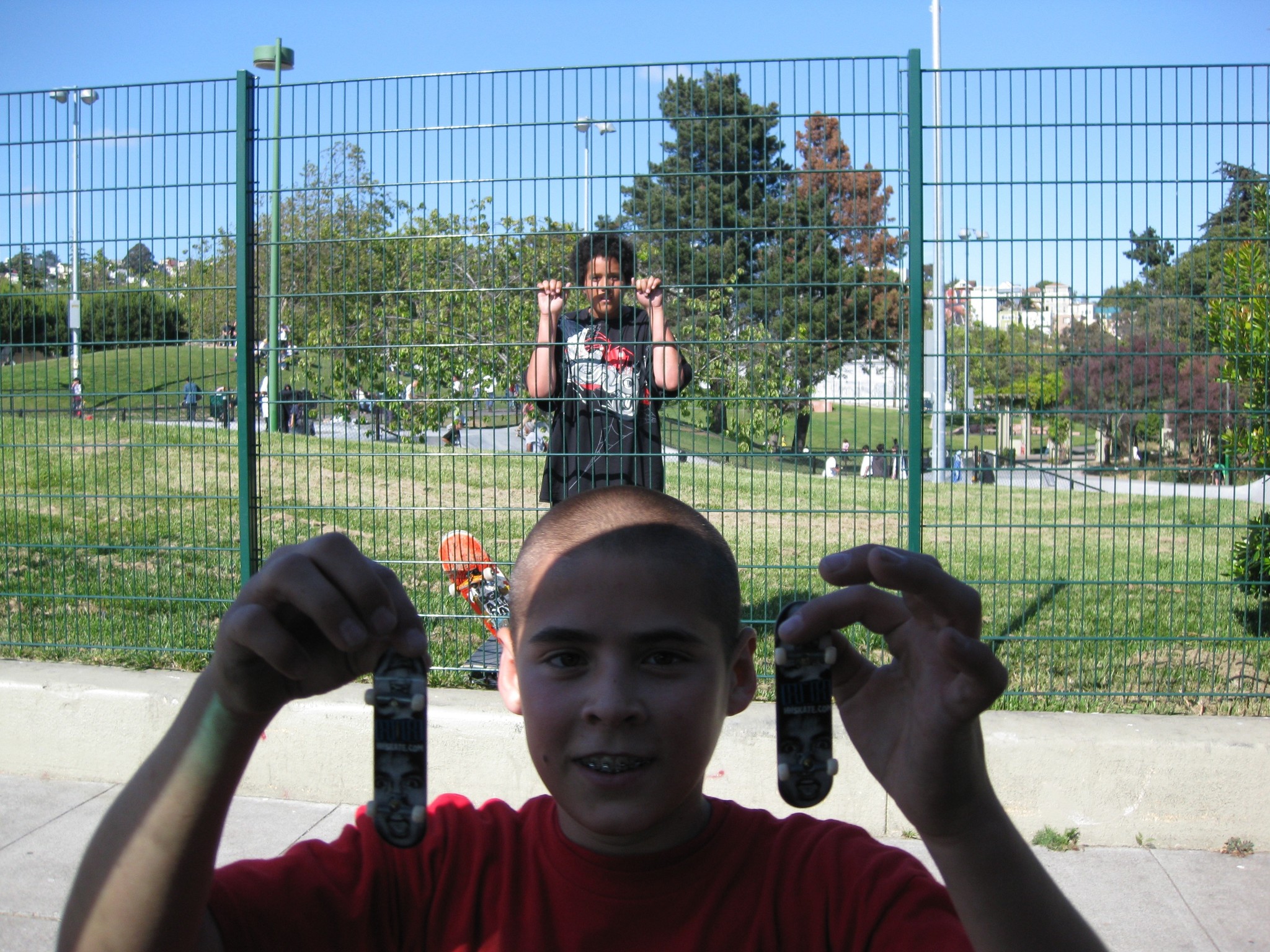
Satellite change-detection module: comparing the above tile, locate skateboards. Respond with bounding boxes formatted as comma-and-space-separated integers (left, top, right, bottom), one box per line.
773, 600, 838, 809
437, 529, 511, 646
364, 667, 429, 848
461, 633, 504, 679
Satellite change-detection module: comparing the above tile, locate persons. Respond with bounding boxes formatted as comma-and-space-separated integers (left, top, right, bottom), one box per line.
781, 434, 787, 451
54, 484, 1111, 952
1210, 464, 1226, 486
181, 376, 198, 422
70, 378, 84, 418
1044, 437, 1050, 455
1, 357, 16, 367
441, 419, 463, 449
526, 233, 692, 508
213, 321, 996, 485
842, 439, 850, 461
678, 449, 688, 463
859, 445, 874, 476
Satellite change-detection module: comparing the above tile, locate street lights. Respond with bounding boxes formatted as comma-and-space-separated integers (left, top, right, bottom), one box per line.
49, 84, 101, 419
252, 35, 294, 431
574, 115, 618, 237
